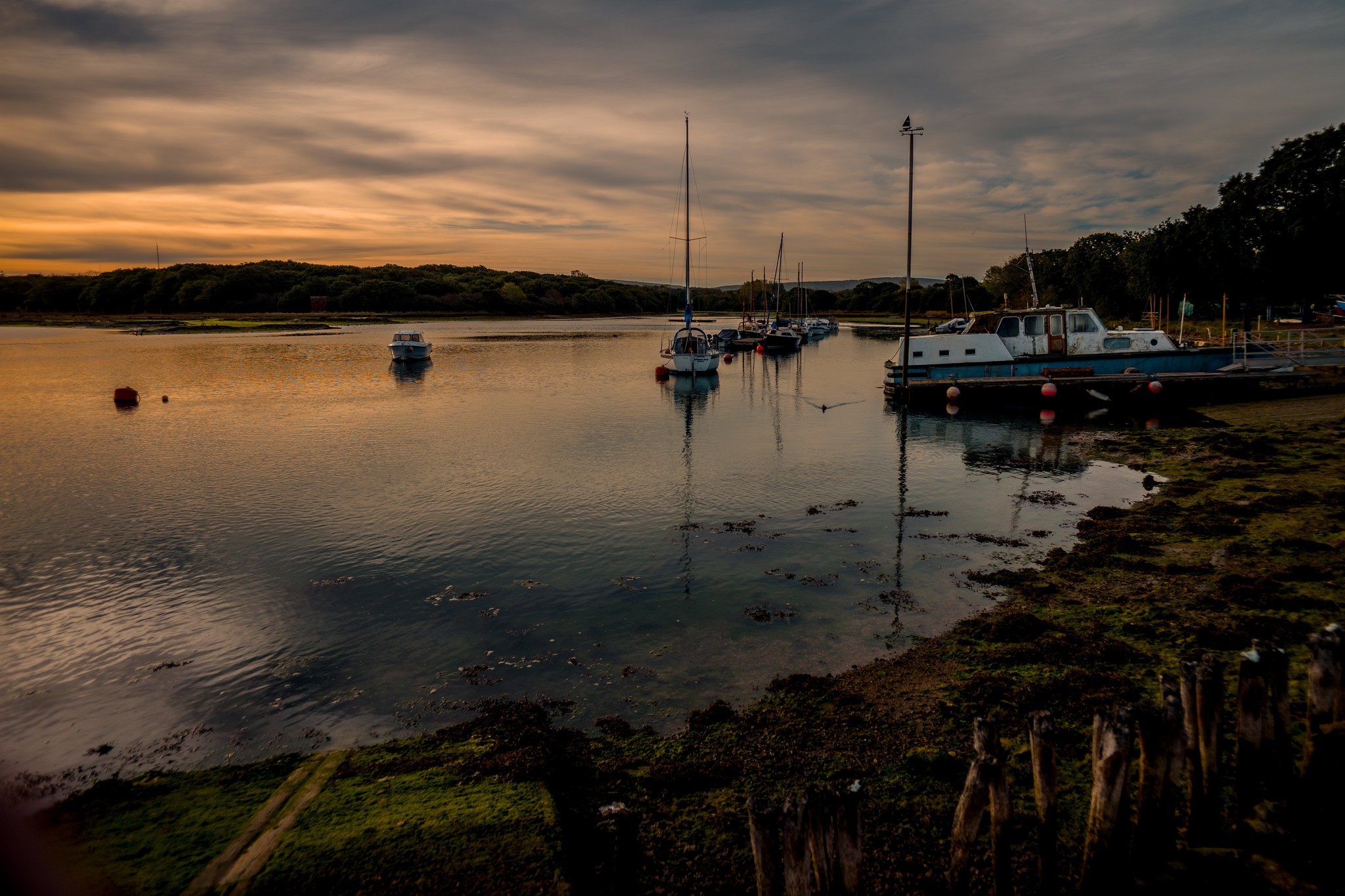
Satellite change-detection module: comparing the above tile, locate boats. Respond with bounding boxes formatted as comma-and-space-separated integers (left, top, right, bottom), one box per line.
388, 330, 433, 364
879, 113, 1267, 409
1333, 304, 1345, 316
1335, 300, 1345, 311
927, 317, 970, 333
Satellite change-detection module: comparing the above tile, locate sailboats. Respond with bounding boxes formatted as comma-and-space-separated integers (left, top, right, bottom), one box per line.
660, 114, 722, 374
704, 230, 839, 353
